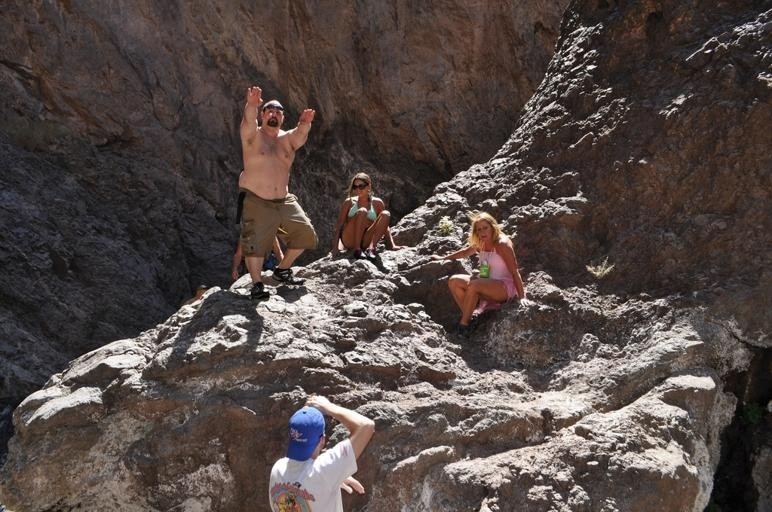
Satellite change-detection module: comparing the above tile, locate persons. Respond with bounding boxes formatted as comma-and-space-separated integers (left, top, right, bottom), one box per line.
269, 396, 375, 512
184, 284, 209, 305
332, 172, 406, 260
231, 170, 284, 281
240, 85, 319, 299
429, 212, 530, 339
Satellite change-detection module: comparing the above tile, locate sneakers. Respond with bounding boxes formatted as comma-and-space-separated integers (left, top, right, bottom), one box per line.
273, 268, 305, 285
354, 248, 376, 260
251, 282, 269, 299
457, 323, 470, 340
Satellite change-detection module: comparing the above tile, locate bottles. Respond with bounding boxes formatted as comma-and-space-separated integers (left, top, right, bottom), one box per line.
480, 261, 489, 278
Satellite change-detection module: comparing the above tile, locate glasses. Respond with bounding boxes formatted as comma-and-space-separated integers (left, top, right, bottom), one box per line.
352, 184, 368, 190
266, 104, 284, 111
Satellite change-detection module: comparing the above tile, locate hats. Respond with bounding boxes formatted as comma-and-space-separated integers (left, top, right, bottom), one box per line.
287, 406, 325, 461
